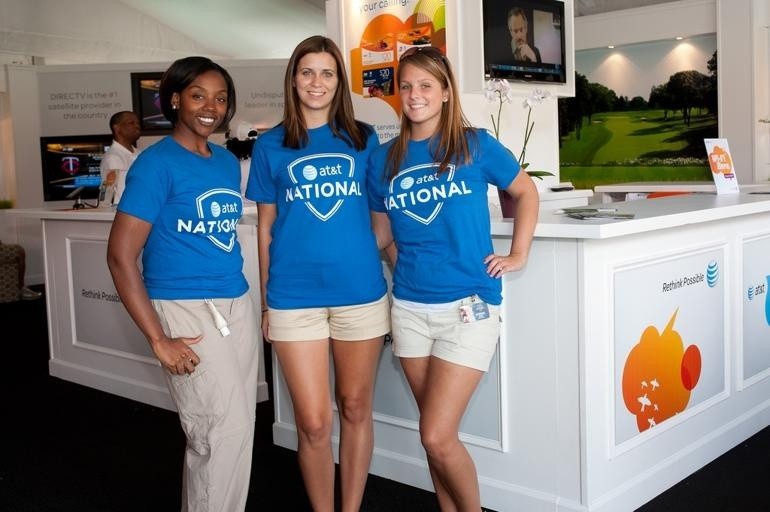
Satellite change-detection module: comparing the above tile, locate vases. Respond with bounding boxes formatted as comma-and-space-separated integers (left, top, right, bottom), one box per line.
497, 187, 516, 217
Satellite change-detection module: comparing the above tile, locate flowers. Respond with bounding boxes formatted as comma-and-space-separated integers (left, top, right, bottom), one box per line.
482, 76, 554, 181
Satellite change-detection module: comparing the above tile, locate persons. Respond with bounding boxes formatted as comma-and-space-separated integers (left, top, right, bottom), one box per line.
365, 48, 540, 511
414, 30, 432, 45
376, 86, 385, 97
98, 111, 144, 205
495, 7, 544, 66
106, 56, 260, 512
244, 34, 399, 511
409, 33, 420, 44
368, 86, 380, 96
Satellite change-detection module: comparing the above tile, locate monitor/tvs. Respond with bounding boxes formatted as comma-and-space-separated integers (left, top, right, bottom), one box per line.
129, 71, 176, 136
38, 134, 114, 202
482, 0, 568, 84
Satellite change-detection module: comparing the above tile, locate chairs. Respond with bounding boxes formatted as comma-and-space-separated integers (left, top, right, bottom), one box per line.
0, 245, 20, 304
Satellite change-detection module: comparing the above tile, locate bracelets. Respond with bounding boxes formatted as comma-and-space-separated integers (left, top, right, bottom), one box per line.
262, 308, 268, 312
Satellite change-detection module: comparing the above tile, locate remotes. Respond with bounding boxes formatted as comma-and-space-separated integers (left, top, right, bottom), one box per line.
551, 186, 573, 192
